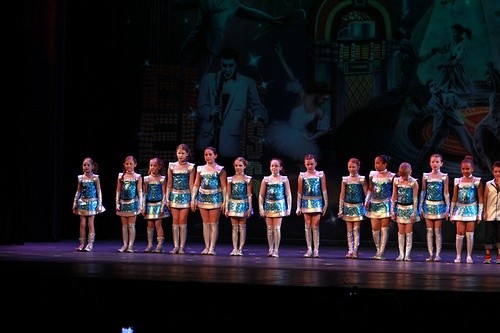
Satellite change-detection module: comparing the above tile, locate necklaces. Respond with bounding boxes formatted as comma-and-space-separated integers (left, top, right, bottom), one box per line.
84, 172, 94, 176
378, 168, 387, 174
272, 172, 280, 177
125, 170, 135, 174
236, 172, 244, 175
432, 169, 441, 174
207, 162, 217, 166
463, 174, 472, 179
307, 170, 317, 173
349, 173, 359, 177
178, 161, 188, 166
151, 173, 160, 177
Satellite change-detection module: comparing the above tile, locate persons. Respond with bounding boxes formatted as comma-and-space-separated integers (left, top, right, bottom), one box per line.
390, 162, 421, 261
483, 161, 500, 264
224, 157, 253, 256
448, 155, 484, 263
337, 158, 370, 258
115, 154, 144, 253
363, 154, 398, 260
140, 157, 167, 253
190, 147, 227, 255
296, 153, 328, 258
418, 154, 451, 262
72, 158, 105, 252
166, 143, 196, 254
258, 158, 292, 258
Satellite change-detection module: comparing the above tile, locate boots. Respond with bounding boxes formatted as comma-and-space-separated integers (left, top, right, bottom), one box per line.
404, 231, 414, 261
303, 223, 312, 256
126, 223, 138, 252
312, 224, 321, 257
272, 224, 282, 256
178, 223, 188, 254
433, 227, 444, 261
237, 223, 248, 255
374, 227, 389, 260
346, 232, 354, 258
395, 232, 404, 260
230, 223, 239, 255
426, 228, 435, 261
464, 232, 475, 263
85, 233, 97, 251
371, 230, 381, 260
118, 224, 129, 252
352, 225, 362, 258
145, 227, 154, 252
266, 224, 274, 256
169, 224, 180, 254
76, 236, 87, 250
208, 221, 220, 255
155, 236, 166, 253
200, 222, 211, 254
454, 233, 466, 263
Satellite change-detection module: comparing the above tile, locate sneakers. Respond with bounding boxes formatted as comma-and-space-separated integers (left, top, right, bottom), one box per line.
495, 255, 500, 263
484, 254, 492, 263
